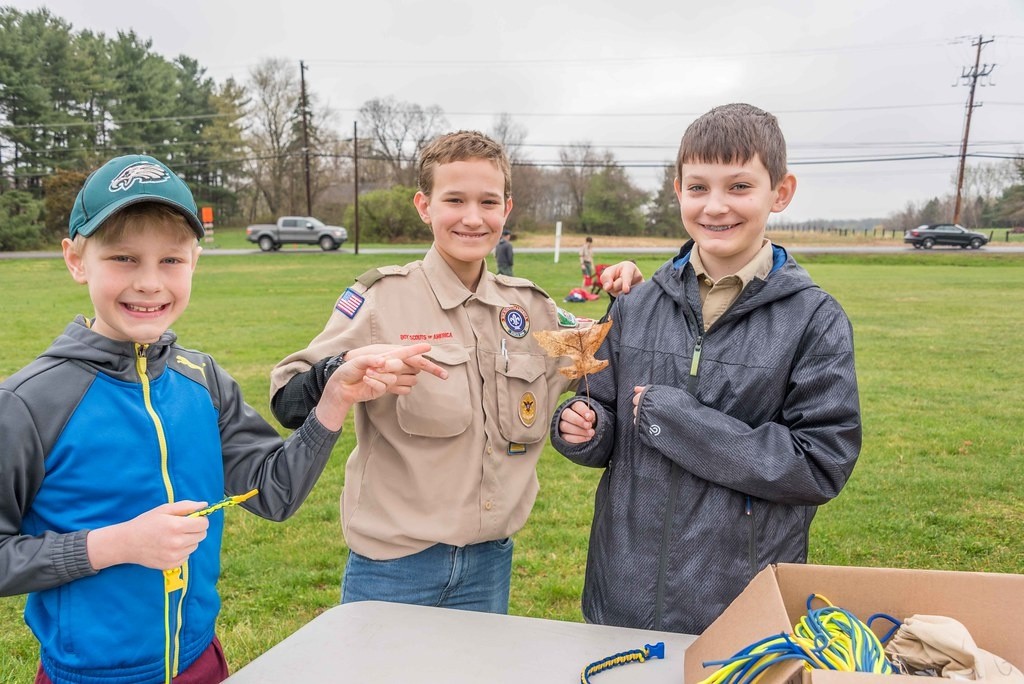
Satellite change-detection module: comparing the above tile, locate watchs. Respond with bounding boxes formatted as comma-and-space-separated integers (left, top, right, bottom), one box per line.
324, 350, 348, 380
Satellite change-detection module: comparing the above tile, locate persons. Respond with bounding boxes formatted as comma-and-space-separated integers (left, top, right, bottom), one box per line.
0, 154, 449, 683
492, 230, 514, 276
269, 131, 645, 616
580, 237, 594, 291
549, 104, 862, 636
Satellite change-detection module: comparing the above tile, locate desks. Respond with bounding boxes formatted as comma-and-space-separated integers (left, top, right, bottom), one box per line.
217, 600, 700, 684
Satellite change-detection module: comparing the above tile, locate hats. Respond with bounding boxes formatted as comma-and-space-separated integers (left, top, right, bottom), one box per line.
504, 231, 512, 235
69, 154, 204, 241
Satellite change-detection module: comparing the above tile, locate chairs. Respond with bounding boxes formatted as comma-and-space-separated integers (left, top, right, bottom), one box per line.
590, 265, 612, 295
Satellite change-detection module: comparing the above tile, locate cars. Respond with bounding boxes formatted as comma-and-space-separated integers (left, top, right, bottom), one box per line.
904, 224, 988, 249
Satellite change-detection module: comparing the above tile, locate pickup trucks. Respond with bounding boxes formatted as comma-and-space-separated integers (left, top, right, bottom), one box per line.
245, 214, 348, 251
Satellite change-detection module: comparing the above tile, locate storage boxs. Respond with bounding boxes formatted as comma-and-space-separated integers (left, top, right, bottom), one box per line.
683, 562, 1024, 684
